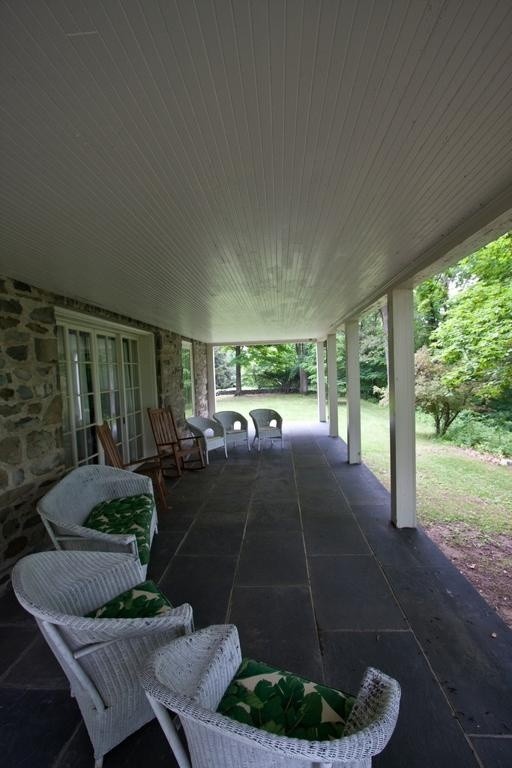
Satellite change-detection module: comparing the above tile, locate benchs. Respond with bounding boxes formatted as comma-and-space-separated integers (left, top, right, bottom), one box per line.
37, 464, 159, 582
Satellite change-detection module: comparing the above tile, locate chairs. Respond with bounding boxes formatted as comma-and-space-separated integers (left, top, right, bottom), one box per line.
213, 410, 251, 458
184, 415, 229, 465
249, 408, 283, 452
11, 548, 194, 767
95, 420, 170, 510
135, 622, 402, 767
147, 404, 205, 477
